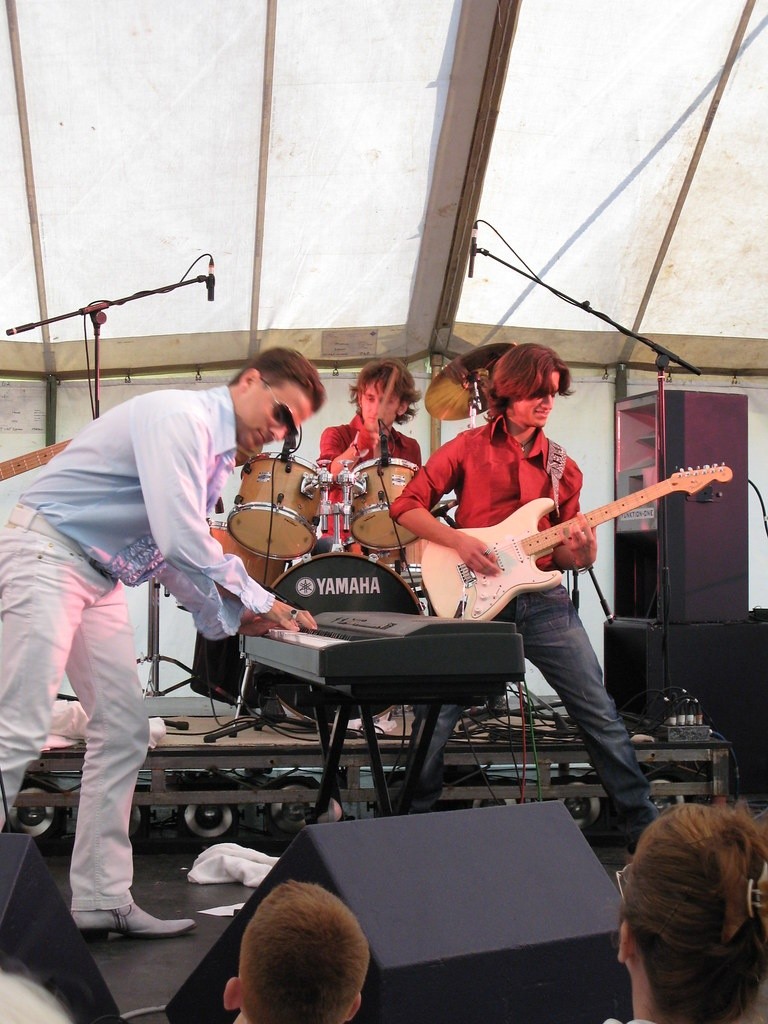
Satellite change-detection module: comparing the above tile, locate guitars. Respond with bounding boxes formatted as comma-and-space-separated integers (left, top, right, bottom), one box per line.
0, 439, 72, 482
421, 462, 733, 620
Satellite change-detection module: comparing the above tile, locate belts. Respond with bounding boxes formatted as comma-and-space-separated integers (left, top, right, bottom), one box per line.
8, 506, 88, 559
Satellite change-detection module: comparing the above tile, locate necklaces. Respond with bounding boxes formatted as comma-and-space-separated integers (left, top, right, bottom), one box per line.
512, 437, 534, 451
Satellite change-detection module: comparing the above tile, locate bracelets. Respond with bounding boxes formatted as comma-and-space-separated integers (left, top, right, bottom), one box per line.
351, 440, 363, 460
570, 554, 594, 574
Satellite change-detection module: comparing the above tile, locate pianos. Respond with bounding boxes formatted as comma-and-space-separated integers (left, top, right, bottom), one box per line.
238, 608, 527, 824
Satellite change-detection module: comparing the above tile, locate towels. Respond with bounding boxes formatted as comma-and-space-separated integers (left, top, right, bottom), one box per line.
45, 700, 167, 750
187, 843, 280, 887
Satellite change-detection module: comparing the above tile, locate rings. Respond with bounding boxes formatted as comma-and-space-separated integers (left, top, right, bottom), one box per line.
482, 548, 493, 557
289, 608, 298, 621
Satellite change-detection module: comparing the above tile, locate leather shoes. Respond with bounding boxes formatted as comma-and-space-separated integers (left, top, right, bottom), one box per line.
70, 899, 197, 941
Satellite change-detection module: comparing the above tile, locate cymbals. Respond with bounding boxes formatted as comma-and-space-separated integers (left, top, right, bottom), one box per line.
424, 341, 516, 422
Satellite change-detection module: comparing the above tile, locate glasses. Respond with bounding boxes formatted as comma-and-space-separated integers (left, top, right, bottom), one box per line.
616, 863, 631, 904
259, 376, 298, 436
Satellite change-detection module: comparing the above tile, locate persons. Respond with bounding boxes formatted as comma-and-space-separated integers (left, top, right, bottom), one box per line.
0, 347, 327, 940
389, 343, 660, 857
602, 801, 768, 1024
223, 880, 370, 1024
311, 358, 421, 555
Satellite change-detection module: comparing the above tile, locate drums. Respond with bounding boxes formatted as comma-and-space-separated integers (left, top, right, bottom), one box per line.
175, 517, 294, 618
226, 448, 325, 559
347, 455, 426, 552
341, 532, 428, 588
258, 552, 427, 729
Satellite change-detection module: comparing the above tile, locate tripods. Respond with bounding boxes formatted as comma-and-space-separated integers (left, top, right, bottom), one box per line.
436, 249, 712, 782
133, 581, 251, 717
204, 691, 356, 743
456, 695, 574, 732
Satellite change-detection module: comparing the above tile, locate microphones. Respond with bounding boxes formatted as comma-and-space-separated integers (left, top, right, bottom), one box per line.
379, 431, 389, 467
432, 500, 458, 517
468, 221, 479, 278
207, 258, 215, 301
280, 439, 292, 462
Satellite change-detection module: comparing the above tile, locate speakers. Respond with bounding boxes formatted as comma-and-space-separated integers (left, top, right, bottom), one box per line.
0, 832, 122, 1024
603, 390, 768, 796
164, 800, 635, 1024
